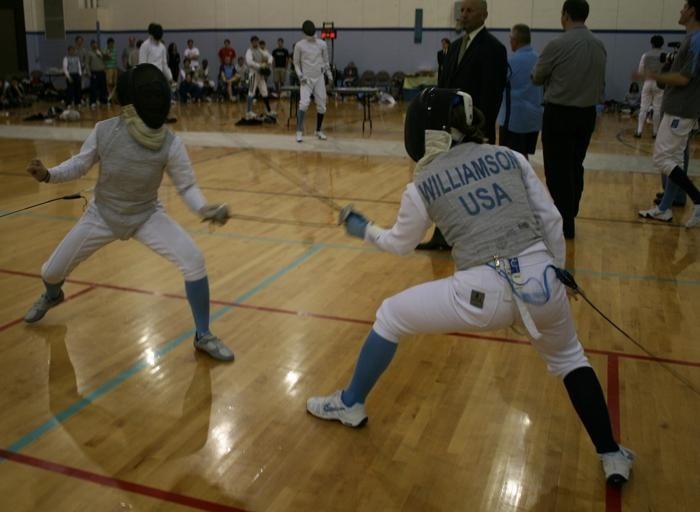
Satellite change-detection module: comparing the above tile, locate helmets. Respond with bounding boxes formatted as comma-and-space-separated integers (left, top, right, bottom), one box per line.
406, 87, 482, 164
117, 64, 177, 150
303, 20, 315, 36
149, 23, 163, 40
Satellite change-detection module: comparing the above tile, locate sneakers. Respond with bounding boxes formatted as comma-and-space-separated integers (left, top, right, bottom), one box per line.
25, 291, 64, 323
247, 112, 256, 119
639, 206, 673, 222
193, 332, 234, 361
314, 132, 327, 140
654, 192, 664, 205
415, 242, 452, 253
307, 390, 368, 428
652, 134, 657, 139
602, 444, 634, 483
685, 204, 700, 227
64, 101, 112, 110
267, 111, 277, 117
297, 132, 303, 143
633, 133, 642, 138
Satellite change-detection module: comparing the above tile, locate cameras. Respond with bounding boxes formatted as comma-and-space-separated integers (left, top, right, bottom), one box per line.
660, 51, 678, 63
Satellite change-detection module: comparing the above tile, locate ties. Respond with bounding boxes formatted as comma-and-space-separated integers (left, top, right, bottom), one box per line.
457, 33, 469, 63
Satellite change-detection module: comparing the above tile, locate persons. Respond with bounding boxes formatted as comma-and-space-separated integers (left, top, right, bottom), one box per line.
24, 64, 234, 362
2, 20, 332, 138
403, 1, 610, 248
308, 86, 637, 492
629, 1, 700, 226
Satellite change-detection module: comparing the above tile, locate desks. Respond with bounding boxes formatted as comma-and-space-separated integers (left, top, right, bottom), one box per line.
280, 86, 377, 132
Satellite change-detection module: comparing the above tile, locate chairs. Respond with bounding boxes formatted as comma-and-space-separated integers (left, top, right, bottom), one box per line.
360, 71, 404, 99
